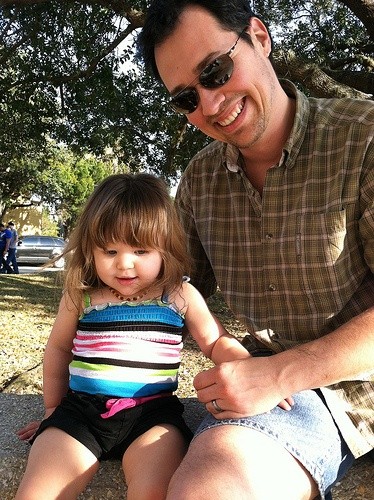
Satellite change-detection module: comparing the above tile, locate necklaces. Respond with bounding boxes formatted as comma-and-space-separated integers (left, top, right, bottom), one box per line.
107, 285, 151, 300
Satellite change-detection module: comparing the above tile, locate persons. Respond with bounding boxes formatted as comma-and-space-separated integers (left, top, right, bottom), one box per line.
134, 0, 374, 500
0, 221, 20, 274
13, 173, 295, 500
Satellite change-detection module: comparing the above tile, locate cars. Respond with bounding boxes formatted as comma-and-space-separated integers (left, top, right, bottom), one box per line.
3, 235, 68, 267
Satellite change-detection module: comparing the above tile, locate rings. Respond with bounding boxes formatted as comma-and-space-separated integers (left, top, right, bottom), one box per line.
211, 400, 225, 413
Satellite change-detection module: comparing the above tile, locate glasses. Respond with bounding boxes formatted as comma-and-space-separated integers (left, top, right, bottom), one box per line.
167, 26, 248, 113
